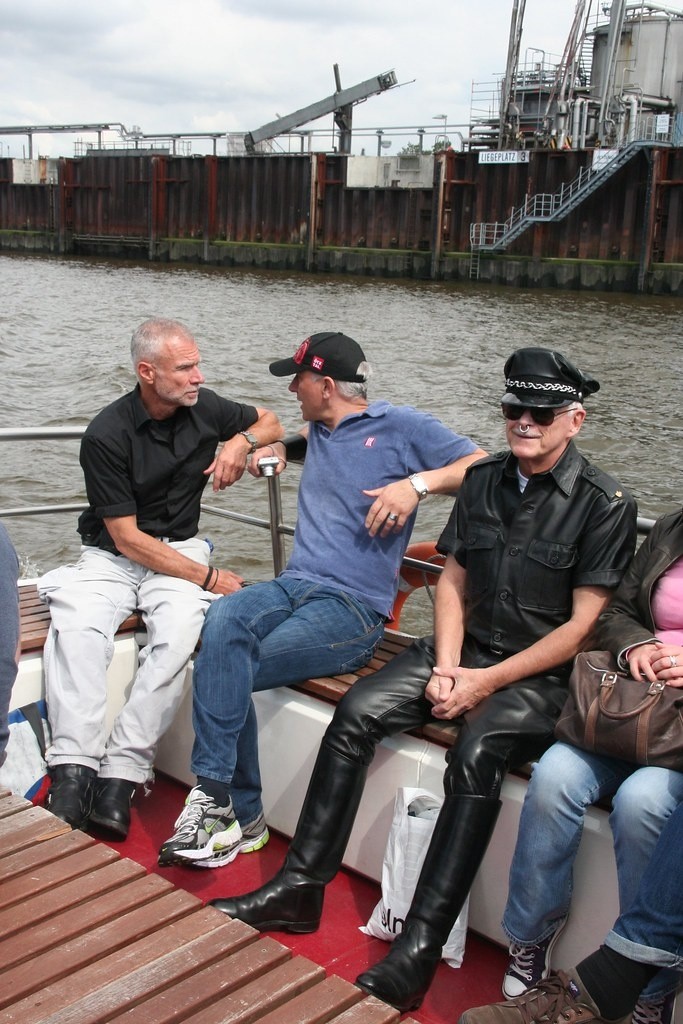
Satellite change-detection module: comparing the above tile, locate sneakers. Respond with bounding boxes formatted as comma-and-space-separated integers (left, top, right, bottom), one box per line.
457, 963, 630, 1024
624, 998, 670, 1024
157, 784, 243, 868
187, 811, 271, 868
501, 917, 571, 1000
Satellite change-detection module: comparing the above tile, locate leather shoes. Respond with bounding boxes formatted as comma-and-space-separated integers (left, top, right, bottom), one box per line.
45, 765, 100, 828
90, 776, 138, 837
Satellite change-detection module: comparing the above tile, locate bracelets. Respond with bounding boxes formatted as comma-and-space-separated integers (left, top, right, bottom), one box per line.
202, 566, 219, 591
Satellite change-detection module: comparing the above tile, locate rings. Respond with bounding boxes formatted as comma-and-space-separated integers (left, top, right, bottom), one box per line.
670, 655, 677, 667
389, 513, 398, 520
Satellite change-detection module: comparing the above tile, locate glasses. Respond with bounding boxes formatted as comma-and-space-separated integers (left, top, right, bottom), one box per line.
499, 402, 577, 427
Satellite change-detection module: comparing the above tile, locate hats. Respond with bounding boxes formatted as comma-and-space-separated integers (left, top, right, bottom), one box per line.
501, 346, 601, 408
269, 331, 370, 383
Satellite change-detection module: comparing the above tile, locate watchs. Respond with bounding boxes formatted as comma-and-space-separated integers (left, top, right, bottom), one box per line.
242, 431, 257, 454
407, 472, 429, 501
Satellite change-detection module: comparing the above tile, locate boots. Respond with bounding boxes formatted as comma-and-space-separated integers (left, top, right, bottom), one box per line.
354, 790, 503, 1009
206, 734, 371, 932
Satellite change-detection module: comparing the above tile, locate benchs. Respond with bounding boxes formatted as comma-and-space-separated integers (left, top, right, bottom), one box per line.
1, 569, 682, 1024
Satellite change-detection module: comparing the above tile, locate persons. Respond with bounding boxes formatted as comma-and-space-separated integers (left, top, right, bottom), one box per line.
208, 345, 638, 1016
501, 507, 683, 1001
36, 315, 284, 836
460, 785, 683, 1024
158, 331, 491, 868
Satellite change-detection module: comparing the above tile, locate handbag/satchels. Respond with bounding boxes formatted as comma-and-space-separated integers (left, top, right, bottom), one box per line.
555, 647, 683, 770
358, 784, 472, 968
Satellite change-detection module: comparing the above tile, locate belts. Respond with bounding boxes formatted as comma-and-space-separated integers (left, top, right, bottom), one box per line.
154, 536, 176, 544
